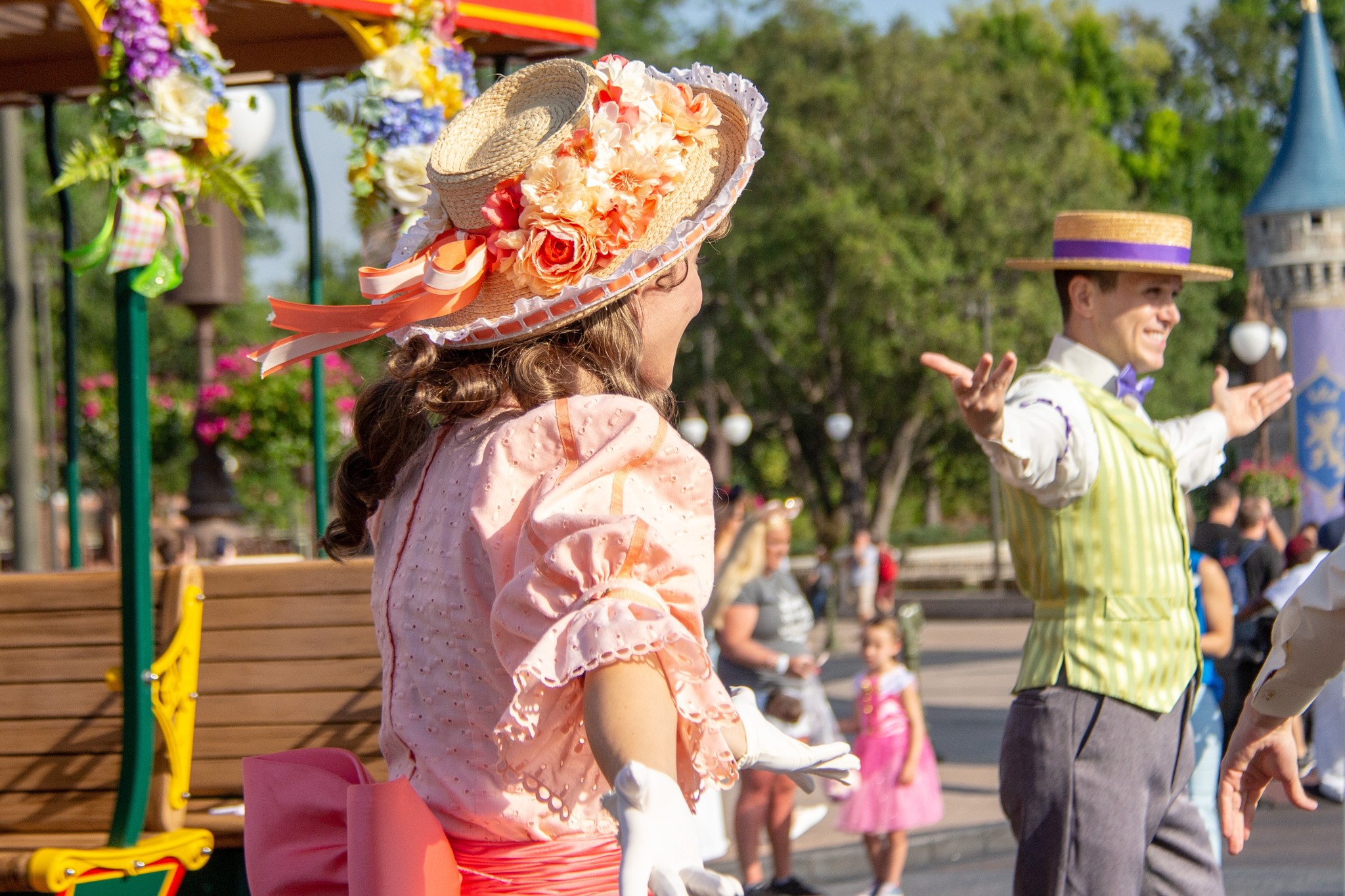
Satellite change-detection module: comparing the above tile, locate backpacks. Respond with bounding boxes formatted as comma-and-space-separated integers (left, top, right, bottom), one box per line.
1220, 537, 1261, 607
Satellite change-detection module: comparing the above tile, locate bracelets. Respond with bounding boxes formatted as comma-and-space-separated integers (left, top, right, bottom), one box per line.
776, 653, 790, 676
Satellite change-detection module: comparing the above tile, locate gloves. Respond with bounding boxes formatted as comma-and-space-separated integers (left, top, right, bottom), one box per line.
600, 761, 746, 896
729, 685, 861, 795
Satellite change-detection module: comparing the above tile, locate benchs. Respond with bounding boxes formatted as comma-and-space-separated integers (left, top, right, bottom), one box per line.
0, 557, 389, 896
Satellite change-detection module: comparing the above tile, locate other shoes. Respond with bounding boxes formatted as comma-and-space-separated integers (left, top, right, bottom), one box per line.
747, 875, 812, 896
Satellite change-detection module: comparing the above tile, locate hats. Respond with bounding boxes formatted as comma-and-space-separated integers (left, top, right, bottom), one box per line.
245, 54, 769, 380
1005, 211, 1234, 281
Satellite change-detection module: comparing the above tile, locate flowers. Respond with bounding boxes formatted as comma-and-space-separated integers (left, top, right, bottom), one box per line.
318, 0, 476, 243
472, 53, 721, 296
44, 0, 266, 292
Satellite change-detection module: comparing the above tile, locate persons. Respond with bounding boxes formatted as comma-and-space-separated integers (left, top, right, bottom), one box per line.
242, 54, 862, 896
838, 614, 946, 896
1192, 481, 1345, 804
1218, 539, 1345, 855
1178, 493, 1233, 867
921, 209, 1293, 896
711, 499, 822, 896
804, 528, 898, 650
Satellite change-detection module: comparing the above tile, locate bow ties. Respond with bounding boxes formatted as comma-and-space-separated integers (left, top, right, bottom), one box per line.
1116, 363, 1158, 403
